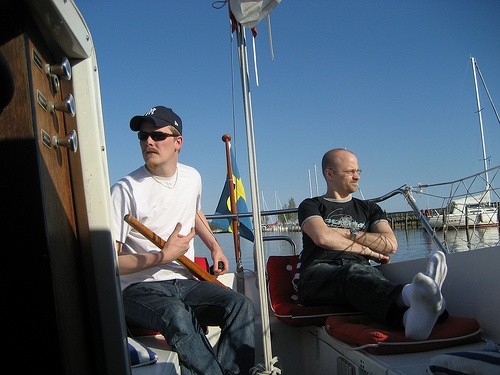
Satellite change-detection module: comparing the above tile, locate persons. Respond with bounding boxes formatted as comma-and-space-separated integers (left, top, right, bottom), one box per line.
298, 148, 449, 340
110, 105, 257, 375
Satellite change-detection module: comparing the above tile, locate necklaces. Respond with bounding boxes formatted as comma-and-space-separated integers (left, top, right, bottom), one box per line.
147, 165, 178, 189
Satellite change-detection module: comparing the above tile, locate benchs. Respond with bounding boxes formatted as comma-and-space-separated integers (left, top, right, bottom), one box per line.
129, 331, 181, 375
268, 255, 500, 375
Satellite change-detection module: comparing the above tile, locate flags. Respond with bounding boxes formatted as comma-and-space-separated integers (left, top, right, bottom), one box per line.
210, 142, 254, 243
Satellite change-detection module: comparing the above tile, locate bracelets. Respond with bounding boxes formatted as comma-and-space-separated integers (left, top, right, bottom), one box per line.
350, 229, 357, 241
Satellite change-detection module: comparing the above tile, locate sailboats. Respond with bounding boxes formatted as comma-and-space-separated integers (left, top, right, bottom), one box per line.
261, 163, 365, 233
420, 52, 500, 233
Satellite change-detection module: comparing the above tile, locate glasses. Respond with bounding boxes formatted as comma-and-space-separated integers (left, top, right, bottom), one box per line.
138, 131, 180, 142
333, 169, 362, 176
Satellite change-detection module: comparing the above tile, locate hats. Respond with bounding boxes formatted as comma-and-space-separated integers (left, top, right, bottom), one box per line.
130, 106, 182, 136
